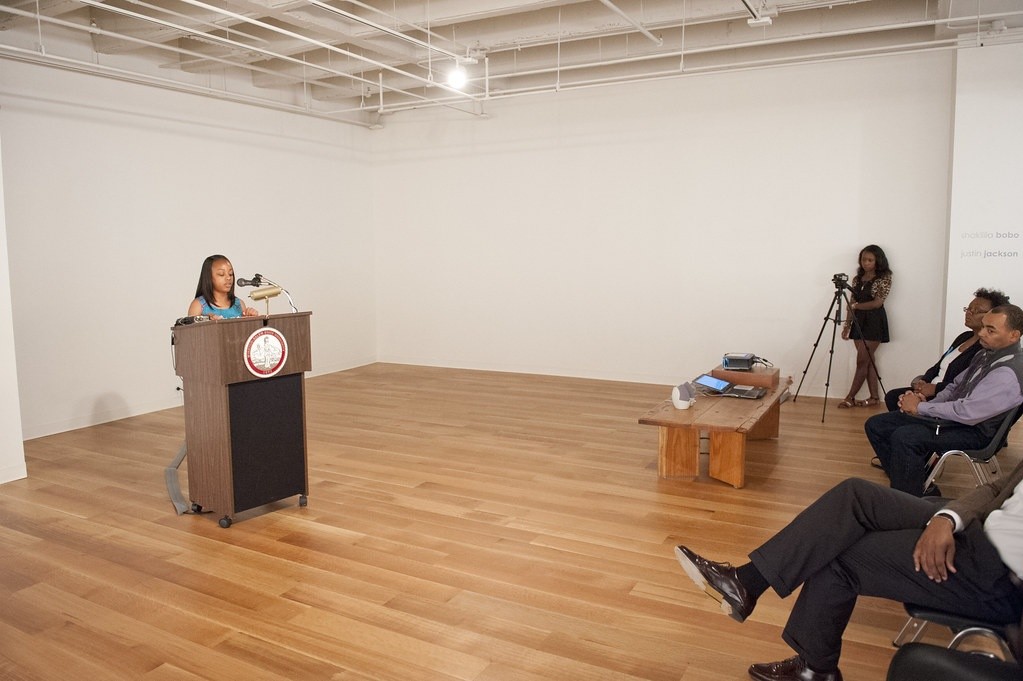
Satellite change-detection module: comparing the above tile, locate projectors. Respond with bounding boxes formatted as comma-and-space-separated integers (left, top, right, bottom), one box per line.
723, 353, 755, 370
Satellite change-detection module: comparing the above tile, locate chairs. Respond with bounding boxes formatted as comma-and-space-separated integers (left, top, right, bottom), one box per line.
886, 642, 1023, 681
892, 597, 1023, 662
919, 405, 1019, 498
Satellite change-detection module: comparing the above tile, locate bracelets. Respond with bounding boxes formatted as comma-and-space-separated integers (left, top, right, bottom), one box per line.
852, 302, 858, 309
935, 515, 954, 528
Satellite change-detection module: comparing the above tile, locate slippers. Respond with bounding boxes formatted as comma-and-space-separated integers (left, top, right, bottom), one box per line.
837, 397, 856, 409
859, 395, 879, 407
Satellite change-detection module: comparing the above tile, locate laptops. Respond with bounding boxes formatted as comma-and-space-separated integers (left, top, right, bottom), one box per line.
692, 374, 767, 399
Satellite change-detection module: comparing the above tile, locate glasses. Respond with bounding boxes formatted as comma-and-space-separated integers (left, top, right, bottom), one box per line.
962, 307, 989, 316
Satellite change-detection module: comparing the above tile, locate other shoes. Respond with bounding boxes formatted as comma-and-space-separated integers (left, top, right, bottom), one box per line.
872, 454, 884, 469
926, 486, 942, 497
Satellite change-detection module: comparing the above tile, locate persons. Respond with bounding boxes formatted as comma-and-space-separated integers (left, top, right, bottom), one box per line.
864, 305, 1023, 498
675, 459, 1023, 681
884, 288, 1012, 412
188, 255, 259, 320
838, 245, 893, 407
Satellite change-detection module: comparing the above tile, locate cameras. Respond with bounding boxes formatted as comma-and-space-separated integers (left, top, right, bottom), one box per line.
832, 273, 848, 282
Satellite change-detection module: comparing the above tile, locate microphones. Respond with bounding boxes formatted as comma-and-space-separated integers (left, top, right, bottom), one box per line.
237, 278, 273, 288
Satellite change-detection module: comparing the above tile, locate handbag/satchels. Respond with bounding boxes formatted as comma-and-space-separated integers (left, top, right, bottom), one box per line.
911, 414, 962, 435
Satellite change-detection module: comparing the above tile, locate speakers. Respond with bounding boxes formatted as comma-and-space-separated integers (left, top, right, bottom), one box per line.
672, 381, 695, 410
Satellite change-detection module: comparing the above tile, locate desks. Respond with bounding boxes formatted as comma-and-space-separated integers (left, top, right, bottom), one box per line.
638, 377, 789, 489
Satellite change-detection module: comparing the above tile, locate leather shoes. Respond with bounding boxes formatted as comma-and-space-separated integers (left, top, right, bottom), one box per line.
749, 655, 842, 681
675, 543, 757, 622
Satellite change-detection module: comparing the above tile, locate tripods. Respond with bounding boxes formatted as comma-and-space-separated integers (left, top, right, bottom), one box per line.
792, 282, 888, 424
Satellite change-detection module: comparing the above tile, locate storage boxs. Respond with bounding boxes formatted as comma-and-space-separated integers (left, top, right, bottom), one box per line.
711, 364, 780, 389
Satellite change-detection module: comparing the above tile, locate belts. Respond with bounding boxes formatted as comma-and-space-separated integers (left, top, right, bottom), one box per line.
1009, 571, 1022, 590
